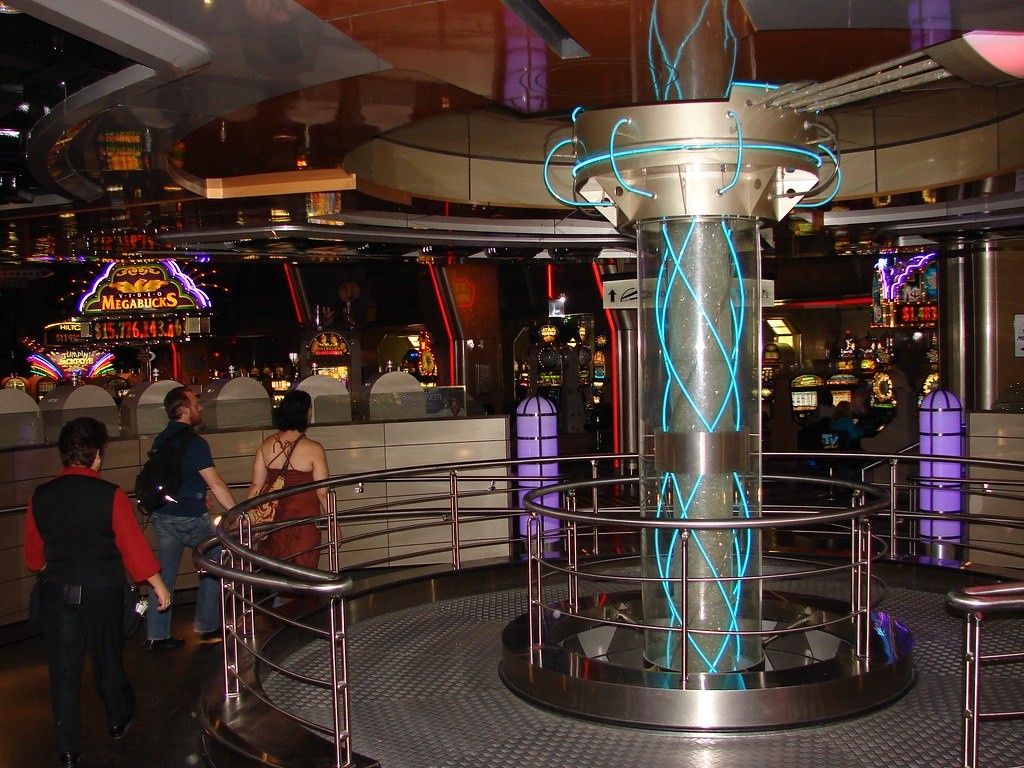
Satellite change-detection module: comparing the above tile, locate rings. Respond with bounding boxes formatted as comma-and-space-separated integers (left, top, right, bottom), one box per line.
168, 601, 171, 605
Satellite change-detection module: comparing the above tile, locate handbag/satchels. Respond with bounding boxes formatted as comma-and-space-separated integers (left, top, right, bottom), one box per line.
230, 474, 285, 542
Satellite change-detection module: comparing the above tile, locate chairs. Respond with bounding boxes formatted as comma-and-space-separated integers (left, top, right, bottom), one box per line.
812, 428, 850, 453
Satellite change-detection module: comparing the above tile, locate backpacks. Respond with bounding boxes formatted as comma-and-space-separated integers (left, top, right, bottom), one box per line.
134, 429, 192, 516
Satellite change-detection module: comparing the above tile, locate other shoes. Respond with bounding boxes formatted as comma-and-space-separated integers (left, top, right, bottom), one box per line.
202, 627, 230, 641
148, 638, 185, 654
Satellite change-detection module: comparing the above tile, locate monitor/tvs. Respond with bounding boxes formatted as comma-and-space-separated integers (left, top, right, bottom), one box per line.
791, 391, 818, 412
831, 390, 852, 407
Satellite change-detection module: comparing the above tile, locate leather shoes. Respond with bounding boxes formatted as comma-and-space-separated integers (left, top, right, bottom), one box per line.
107, 714, 134, 739
59, 747, 84, 768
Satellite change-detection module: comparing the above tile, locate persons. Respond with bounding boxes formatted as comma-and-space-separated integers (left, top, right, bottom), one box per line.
144, 387, 242, 649
798, 390, 898, 488
21, 417, 172, 768
247, 391, 343, 608
